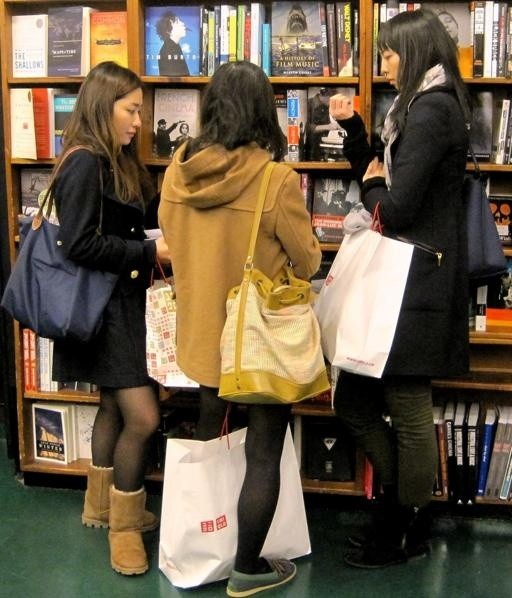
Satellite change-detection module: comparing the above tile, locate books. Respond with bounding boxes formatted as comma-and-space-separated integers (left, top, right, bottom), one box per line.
10, 1, 512, 505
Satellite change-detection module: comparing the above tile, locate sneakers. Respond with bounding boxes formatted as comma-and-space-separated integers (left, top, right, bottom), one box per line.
226, 556, 297, 598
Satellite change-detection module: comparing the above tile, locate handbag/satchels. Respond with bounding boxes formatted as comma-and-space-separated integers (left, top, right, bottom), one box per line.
312, 228, 414, 376
1, 214, 119, 342
218, 266, 332, 404
158, 423, 312, 589
145, 276, 200, 388
456, 177, 509, 288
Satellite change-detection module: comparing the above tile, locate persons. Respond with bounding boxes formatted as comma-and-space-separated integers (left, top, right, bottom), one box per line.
51, 61, 173, 576
157, 60, 322, 598
330, 9, 468, 570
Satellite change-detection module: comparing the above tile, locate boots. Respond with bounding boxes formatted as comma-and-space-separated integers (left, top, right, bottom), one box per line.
108, 483, 150, 576
344, 482, 433, 569
81, 460, 159, 533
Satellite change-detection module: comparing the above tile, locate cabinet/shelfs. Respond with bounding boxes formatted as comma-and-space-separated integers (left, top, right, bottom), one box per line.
1, 2, 510, 505
